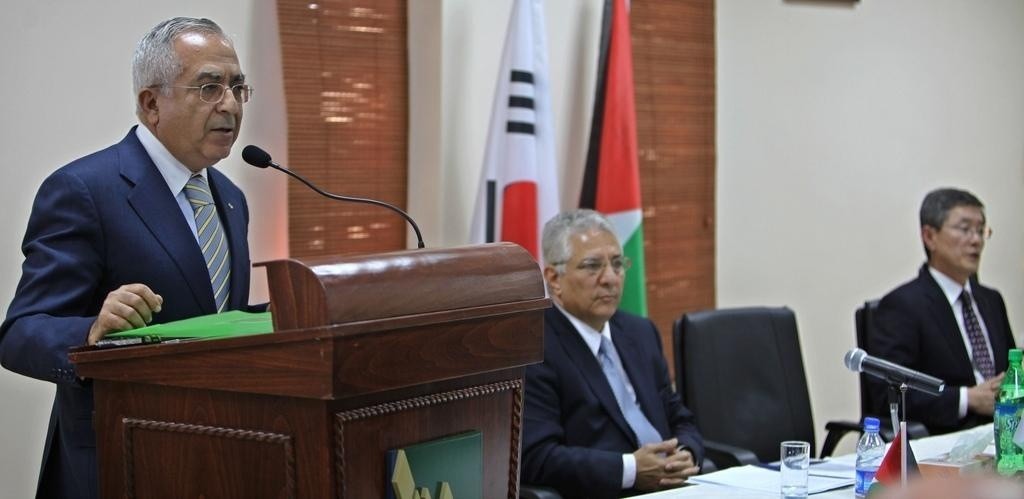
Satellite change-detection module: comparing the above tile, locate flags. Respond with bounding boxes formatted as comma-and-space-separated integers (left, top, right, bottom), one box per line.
469, 0, 560, 298
577, 0, 647, 317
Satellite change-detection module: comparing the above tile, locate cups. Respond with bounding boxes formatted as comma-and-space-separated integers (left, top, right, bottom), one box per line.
778, 441, 811, 499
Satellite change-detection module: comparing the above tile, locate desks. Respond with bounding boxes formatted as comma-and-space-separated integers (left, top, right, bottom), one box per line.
638, 417, 1024, 498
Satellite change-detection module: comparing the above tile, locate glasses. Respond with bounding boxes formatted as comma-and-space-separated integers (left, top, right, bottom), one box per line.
942, 223, 993, 240
159, 82, 251, 105
569, 255, 632, 275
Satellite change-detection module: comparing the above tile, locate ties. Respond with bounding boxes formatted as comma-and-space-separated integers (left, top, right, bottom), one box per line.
958, 290, 996, 379
600, 335, 666, 457
183, 174, 231, 313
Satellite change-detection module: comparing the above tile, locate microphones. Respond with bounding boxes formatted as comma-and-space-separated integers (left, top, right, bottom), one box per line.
240, 144, 425, 248
843, 343, 946, 398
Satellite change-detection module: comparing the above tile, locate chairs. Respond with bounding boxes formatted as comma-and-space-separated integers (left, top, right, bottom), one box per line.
851, 306, 930, 440
670, 304, 878, 466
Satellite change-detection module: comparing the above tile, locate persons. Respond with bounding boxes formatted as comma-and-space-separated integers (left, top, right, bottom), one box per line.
869, 188, 1016, 435
517, 209, 707, 493
1, 17, 271, 499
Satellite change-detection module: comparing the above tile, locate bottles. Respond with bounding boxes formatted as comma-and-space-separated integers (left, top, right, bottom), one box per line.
854, 415, 887, 499
994, 347, 1024, 478
